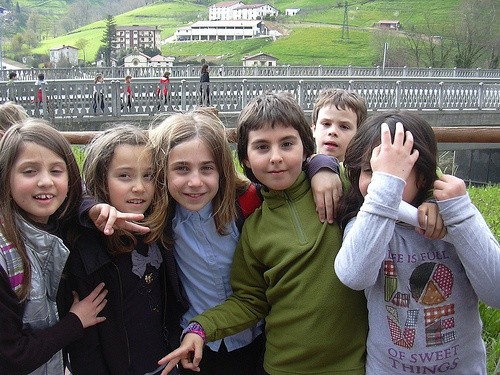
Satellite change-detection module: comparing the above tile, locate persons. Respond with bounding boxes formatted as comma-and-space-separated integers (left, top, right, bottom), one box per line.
77, 107, 344, 375
0, 118, 109, 375
55, 124, 194, 375
92, 74, 105, 113
120, 76, 133, 112
34, 73, 50, 113
198, 64, 212, 106
0, 100, 29, 132
7, 73, 18, 103
309, 89, 368, 162
159, 94, 447, 375
333, 111, 500, 375
158, 71, 172, 109
